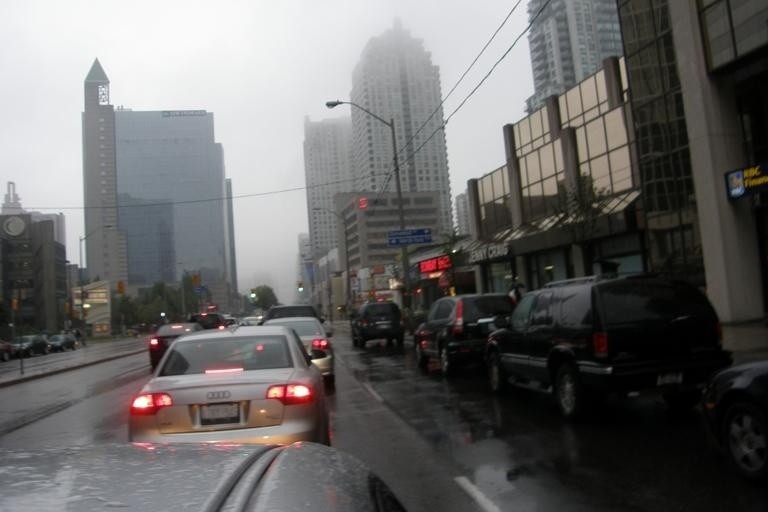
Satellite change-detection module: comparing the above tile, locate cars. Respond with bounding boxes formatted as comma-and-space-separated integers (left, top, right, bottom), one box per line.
0, 442, 405, 512
0, 327, 86, 362
694, 349, 768, 479
148, 302, 324, 365
261, 316, 334, 383
128, 325, 331, 448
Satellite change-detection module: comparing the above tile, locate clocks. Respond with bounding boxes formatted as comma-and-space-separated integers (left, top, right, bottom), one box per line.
3, 216, 26, 237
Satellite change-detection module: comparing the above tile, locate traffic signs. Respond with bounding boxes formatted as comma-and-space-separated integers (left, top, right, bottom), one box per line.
385, 227, 432, 246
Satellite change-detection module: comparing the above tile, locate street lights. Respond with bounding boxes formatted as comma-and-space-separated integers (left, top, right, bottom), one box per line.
78, 224, 113, 345
325, 100, 415, 335
312, 206, 351, 306
305, 243, 334, 323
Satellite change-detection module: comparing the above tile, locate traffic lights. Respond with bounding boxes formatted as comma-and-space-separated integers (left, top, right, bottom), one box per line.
251, 288, 256, 299
298, 282, 304, 293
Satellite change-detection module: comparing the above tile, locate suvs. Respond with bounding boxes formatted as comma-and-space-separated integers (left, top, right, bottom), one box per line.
348, 300, 406, 345
413, 292, 517, 374
484, 272, 723, 416
